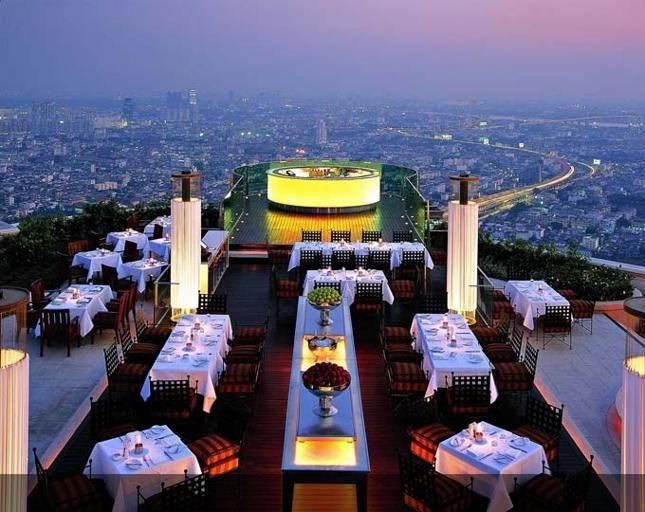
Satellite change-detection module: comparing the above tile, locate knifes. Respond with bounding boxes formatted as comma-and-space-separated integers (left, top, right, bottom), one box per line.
509, 445, 529, 454
460, 444, 472, 452
465, 350, 480, 353
456, 332, 471, 334
163, 452, 172, 459
478, 452, 493, 461
490, 429, 502, 435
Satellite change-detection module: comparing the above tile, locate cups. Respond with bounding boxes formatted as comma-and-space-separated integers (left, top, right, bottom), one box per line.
112, 453, 121, 460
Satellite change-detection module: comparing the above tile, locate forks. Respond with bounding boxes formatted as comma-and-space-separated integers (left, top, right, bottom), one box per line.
497, 452, 514, 461
505, 453, 516, 459
455, 437, 460, 447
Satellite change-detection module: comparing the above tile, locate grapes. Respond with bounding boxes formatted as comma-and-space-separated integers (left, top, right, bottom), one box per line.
302, 361, 349, 386
307, 286, 341, 304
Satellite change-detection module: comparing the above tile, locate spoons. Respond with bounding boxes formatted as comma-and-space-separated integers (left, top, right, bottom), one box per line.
141, 431, 151, 440
143, 458, 150, 467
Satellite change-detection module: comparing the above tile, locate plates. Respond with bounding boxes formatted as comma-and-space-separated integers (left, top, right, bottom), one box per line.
420, 311, 483, 367
495, 453, 515, 463
127, 432, 142, 438
481, 427, 494, 433
168, 447, 181, 454
152, 426, 162, 433
51, 285, 105, 306
126, 459, 142, 469
519, 281, 558, 302
507, 436, 529, 447
448, 438, 466, 447
165, 313, 224, 368
317, 267, 384, 283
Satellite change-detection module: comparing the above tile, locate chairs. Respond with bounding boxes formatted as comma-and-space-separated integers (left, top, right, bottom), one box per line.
265, 228, 425, 333
